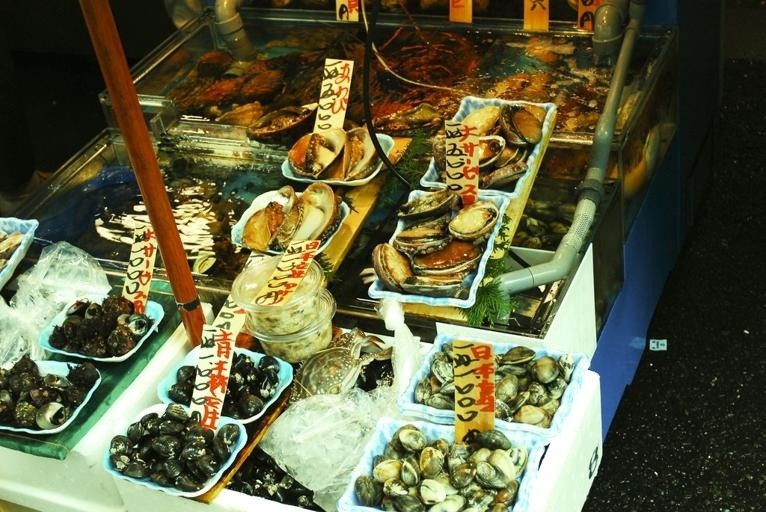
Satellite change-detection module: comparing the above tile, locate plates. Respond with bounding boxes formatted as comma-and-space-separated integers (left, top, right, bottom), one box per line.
0, 97, 590, 511
